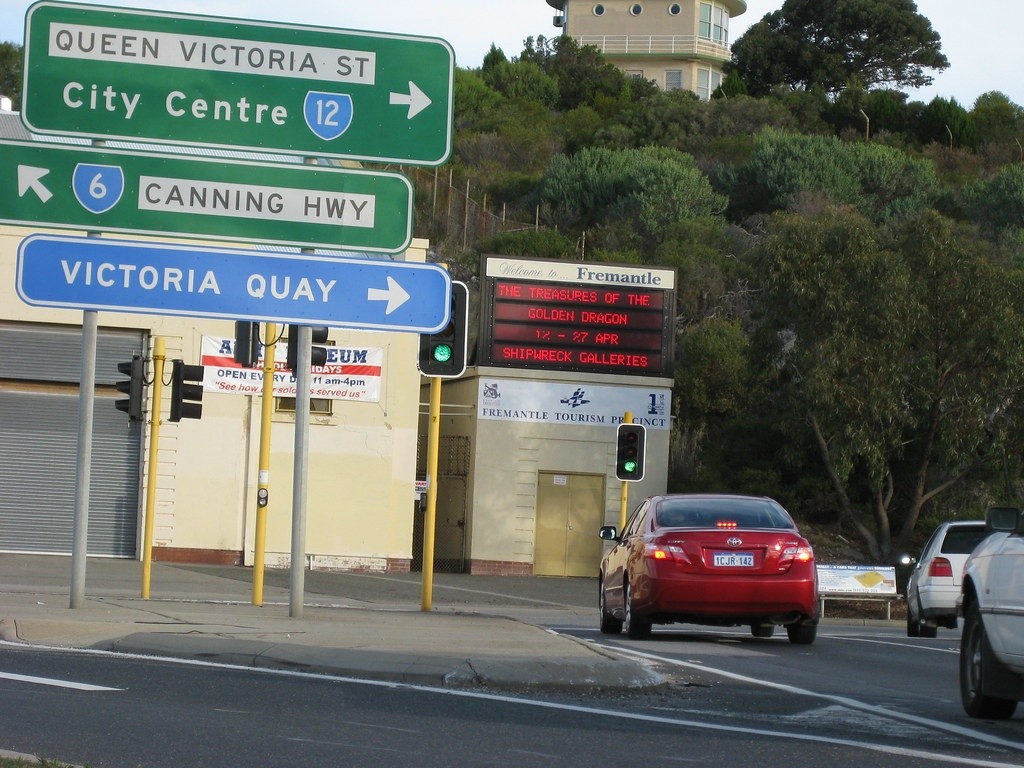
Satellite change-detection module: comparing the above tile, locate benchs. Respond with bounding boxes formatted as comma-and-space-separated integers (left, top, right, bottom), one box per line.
816, 564, 903, 620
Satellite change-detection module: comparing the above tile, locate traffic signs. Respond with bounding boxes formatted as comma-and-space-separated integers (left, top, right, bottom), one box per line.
0, 137, 413, 254
16, 234, 452, 337
19, 0, 455, 169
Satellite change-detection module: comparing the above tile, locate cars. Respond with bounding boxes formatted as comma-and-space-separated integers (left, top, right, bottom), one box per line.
957, 504, 1024, 721
597, 494, 821, 645
899, 520, 992, 639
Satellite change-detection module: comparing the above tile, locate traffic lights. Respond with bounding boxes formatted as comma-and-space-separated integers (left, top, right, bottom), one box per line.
615, 422, 647, 482
170, 360, 205, 425
288, 324, 330, 371
115, 353, 145, 420
417, 280, 471, 380
